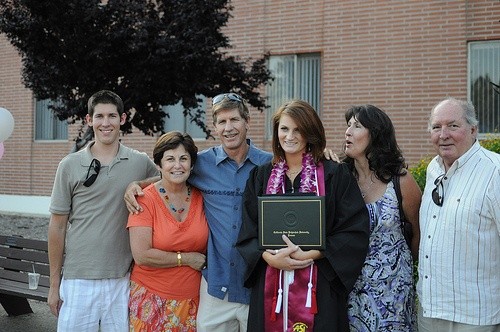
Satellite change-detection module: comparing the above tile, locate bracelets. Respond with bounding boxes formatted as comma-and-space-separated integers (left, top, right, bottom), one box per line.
177, 251, 182, 267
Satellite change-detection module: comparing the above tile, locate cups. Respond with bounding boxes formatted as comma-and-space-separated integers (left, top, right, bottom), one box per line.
28, 273, 40, 290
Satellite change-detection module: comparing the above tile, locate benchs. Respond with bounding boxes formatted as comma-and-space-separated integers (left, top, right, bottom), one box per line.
0, 234, 66, 316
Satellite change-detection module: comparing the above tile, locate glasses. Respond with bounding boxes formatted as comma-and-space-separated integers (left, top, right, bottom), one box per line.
211, 93, 244, 110
83, 159, 101, 187
432, 174, 445, 207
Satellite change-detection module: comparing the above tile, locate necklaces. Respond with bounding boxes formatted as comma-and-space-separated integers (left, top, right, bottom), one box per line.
358, 178, 375, 198
158, 180, 191, 213
286, 170, 299, 175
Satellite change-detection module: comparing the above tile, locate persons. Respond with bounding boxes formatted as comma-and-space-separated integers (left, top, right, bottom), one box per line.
123, 94, 341, 332
342, 104, 423, 332
47, 90, 162, 332
415, 97, 500, 332
236, 99, 370, 332
125, 131, 210, 332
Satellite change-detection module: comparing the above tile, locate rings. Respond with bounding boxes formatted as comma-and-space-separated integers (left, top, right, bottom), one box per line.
275, 249, 278, 255
